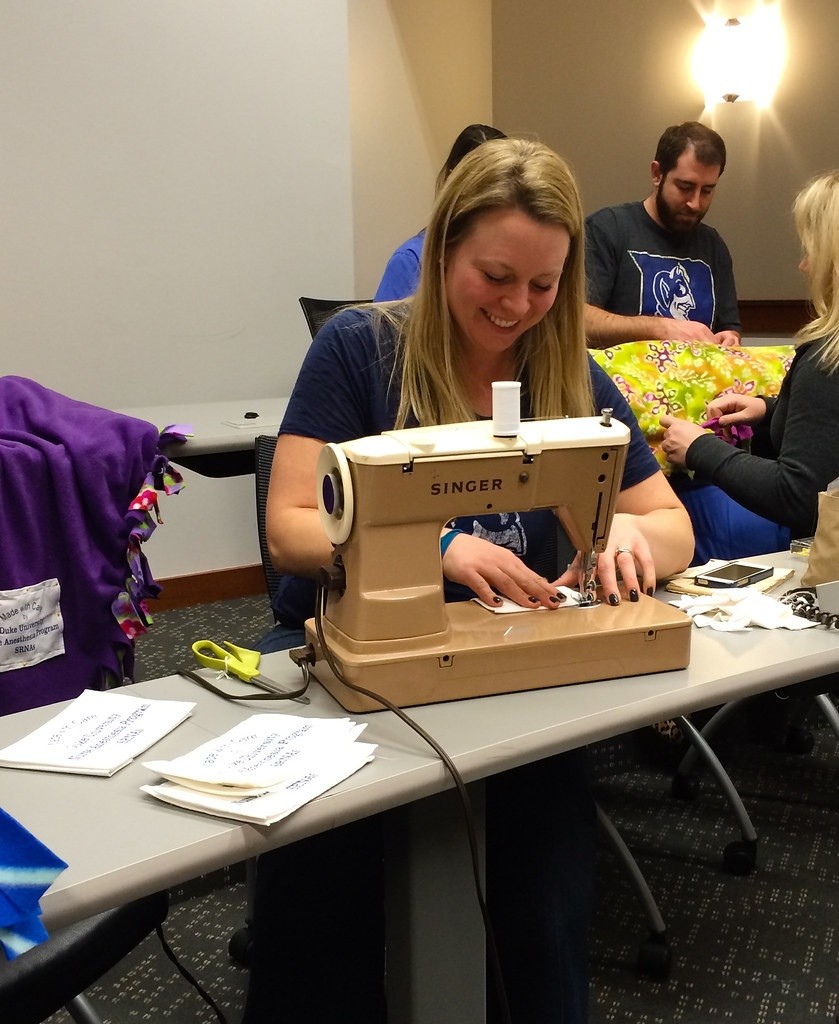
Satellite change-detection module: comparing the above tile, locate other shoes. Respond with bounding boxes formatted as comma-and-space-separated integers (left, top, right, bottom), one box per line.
228, 927, 254, 961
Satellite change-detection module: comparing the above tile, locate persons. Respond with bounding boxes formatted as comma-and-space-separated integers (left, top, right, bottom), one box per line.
240, 138, 694, 1024
377, 125, 503, 303
583, 122, 741, 348
659, 168, 839, 567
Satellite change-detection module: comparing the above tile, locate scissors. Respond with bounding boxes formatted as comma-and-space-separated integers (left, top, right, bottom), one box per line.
192, 640, 309, 705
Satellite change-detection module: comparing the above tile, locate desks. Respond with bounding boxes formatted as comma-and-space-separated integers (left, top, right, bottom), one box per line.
103, 398, 292, 478
0, 549, 839, 1024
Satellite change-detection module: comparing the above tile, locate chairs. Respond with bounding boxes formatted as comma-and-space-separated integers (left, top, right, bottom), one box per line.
671, 690, 839, 801
0, 897, 230, 1024
254, 434, 760, 981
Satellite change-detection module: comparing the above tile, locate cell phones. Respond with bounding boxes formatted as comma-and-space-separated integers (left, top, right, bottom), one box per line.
694, 560, 774, 587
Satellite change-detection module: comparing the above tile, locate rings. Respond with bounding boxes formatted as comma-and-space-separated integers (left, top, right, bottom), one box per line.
617, 547, 634, 559
